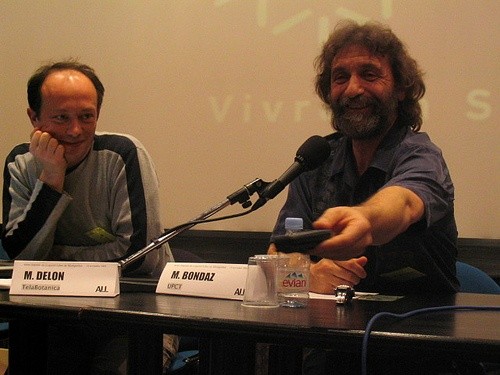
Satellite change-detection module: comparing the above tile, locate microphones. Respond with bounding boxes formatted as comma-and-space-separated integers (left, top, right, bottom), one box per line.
251, 135, 330, 212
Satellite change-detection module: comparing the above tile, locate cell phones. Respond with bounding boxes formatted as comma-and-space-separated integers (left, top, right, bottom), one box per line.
272, 229, 332, 255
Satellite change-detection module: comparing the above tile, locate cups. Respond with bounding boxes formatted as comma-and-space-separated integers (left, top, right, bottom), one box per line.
254, 254, 290, 269
242, 257, 279, 309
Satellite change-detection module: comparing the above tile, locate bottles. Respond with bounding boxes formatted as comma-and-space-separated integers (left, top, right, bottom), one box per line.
277, 218, 310, 308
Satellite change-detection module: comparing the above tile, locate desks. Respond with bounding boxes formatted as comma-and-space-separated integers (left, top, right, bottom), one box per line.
0, 292, 500, 374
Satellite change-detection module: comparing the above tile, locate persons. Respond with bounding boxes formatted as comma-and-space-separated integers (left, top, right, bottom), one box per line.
0, 58, 176, 282
266, 21, 460, 300
